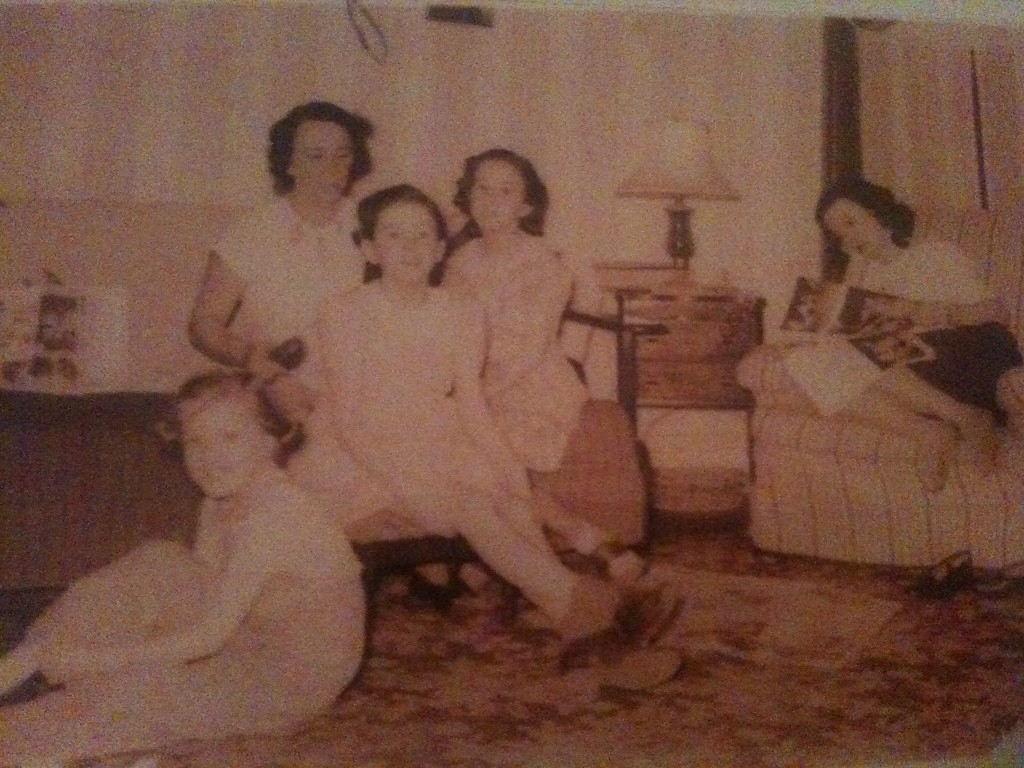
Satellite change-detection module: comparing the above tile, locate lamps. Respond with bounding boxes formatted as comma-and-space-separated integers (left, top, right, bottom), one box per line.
617, 112, 746, 290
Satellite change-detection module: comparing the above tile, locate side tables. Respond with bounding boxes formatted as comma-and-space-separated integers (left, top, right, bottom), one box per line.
615, 277, 767, 533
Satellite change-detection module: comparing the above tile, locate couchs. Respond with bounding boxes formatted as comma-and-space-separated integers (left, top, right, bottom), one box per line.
522, 249, 650, 554
739, 196, 1021, 577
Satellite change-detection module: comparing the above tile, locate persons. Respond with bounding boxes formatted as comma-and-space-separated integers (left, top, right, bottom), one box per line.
441, 148, 658, 596
808, 178, 1022, 496
300, 185, 680, 673
0, 368, 370, 766
189, 99, 452, 607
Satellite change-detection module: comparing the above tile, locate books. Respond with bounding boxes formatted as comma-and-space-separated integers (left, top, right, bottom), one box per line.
755, 272, 937, 417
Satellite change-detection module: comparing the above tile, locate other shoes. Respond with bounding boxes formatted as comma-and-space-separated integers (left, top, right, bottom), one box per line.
551, 581, 626, 655
612, 580, 684, 646
